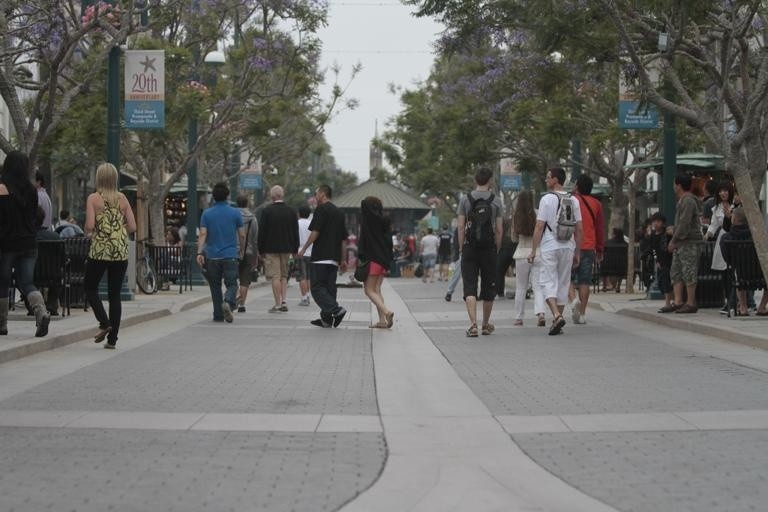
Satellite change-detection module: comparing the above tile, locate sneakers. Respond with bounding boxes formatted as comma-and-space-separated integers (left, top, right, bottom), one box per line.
445, 291, 451, 301
103, 344, 115, 349
94, 326, 113, 343
657, 303, 768, 317
213, 297, 394, 329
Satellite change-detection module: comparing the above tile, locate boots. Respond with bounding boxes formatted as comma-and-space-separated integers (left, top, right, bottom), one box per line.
0, 297, 9, 335
26, 290, 50, 337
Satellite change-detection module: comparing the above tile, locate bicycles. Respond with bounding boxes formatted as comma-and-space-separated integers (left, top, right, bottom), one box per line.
133, 236, 156, 295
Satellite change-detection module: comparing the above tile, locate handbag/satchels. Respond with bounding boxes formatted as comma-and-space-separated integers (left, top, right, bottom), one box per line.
354, 259, 370, 282
242, 253, 257, 267
414, 262, 423, 278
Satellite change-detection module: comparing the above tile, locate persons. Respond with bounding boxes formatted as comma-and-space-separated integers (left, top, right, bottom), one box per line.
0, 150, 137, 349
166, 182, 454, 328
445, 168, 768, 337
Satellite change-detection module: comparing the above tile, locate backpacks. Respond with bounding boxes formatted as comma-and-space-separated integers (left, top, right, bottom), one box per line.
462, 193, 497, 268
540, 192, 577, 243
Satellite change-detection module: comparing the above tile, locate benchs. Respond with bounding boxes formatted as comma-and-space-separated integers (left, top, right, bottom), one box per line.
145, 244, 192, 293
723, 240, 768, 318
695, 242, 725, 307
61, 239, 91, 311
8, 240, 71, 317
573, 245, 641, 293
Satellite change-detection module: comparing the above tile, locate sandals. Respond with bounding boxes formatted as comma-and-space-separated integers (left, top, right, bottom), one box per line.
465, 297, 586, 337
